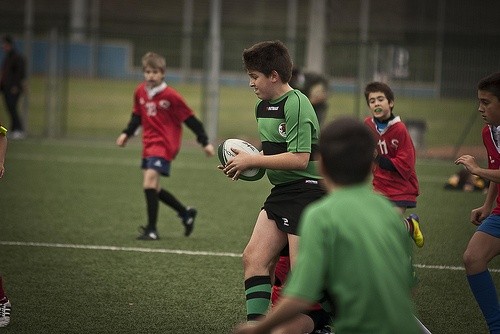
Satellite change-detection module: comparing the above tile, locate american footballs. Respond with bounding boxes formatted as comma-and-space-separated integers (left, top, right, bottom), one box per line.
217, 139, 265, 180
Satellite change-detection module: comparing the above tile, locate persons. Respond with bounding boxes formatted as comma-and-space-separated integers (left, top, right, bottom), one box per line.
362, 81, 424, 250
230, 116, 424, 334
452, 70, 500, 334
0, 34, 27, 140
116, 50, 217, 242
0, 124, 11, 328
215, 38, 335, 334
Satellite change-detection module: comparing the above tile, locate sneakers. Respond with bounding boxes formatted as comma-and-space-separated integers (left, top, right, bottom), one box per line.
136, 226, 160, 240
0, 300, 11, 327
406, 213, 424, 248
183, 207, 197, 236
